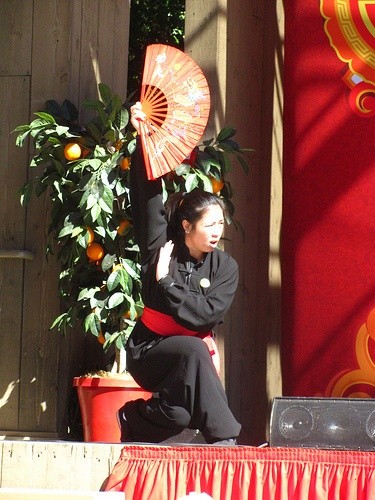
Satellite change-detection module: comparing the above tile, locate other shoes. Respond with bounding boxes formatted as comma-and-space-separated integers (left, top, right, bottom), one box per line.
117, 405, 142, 443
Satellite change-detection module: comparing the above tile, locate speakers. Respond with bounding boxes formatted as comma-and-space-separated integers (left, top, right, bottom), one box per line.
267, 397, 375, 454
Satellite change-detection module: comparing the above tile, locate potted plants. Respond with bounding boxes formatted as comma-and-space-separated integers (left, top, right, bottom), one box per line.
8, 80, 256, 444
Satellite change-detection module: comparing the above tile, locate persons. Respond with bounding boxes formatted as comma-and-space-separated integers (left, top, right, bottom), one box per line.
116, 100, 243, 448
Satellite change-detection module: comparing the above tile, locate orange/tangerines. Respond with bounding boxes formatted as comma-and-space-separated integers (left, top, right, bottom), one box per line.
63, 136, 225, 344
